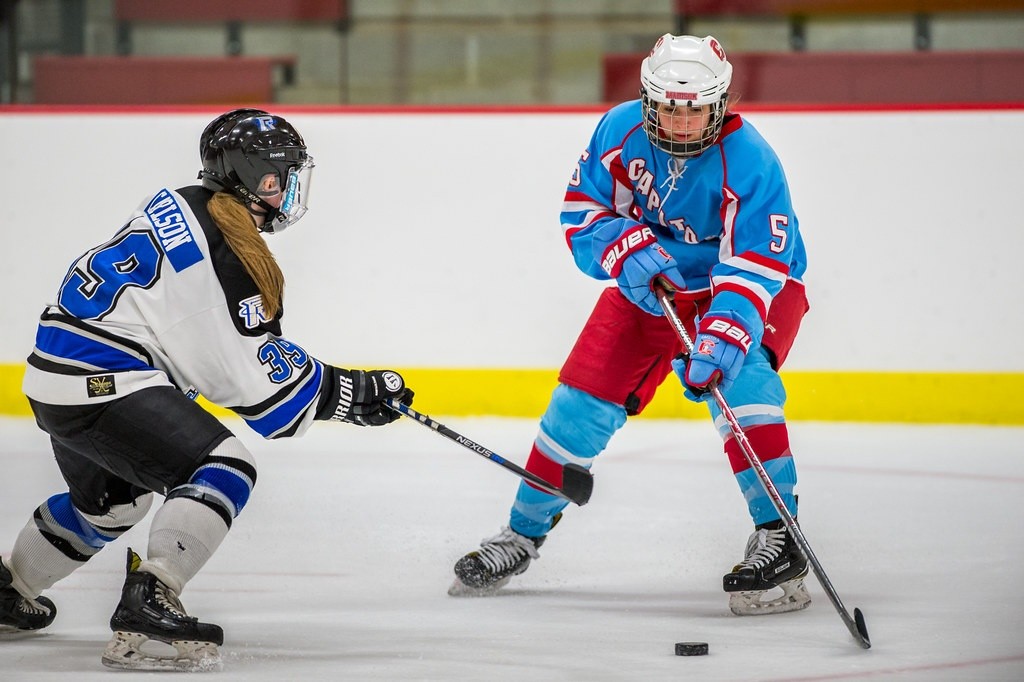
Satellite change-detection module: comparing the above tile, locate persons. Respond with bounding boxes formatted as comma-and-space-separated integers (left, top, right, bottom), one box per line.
455, 33, 809, 593
0, 108, 415, 646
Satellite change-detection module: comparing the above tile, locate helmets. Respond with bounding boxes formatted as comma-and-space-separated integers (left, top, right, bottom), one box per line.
641, 32, 733, 156
199, 110, 315, 233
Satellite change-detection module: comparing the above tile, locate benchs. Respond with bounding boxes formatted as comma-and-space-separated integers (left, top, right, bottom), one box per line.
32, 0, 350, 104
600, 0, 1023, 100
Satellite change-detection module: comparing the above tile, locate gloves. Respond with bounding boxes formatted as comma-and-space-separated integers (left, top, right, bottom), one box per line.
314, 365, 414, 425
670, 310, 752, 403
615, 243, 688, 317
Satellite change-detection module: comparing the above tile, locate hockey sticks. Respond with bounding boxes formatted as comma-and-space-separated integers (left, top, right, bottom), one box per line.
654, 280, 873, 652
380, 397, 595, 508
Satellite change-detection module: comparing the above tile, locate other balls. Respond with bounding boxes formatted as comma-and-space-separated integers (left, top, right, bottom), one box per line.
674, 641, 710, 657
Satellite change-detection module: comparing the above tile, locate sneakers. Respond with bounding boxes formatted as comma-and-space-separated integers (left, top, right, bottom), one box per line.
722, 494, 813, 615
0, 558, 58, 639
101, 547, 225, 672
448, 511, 565, 596
